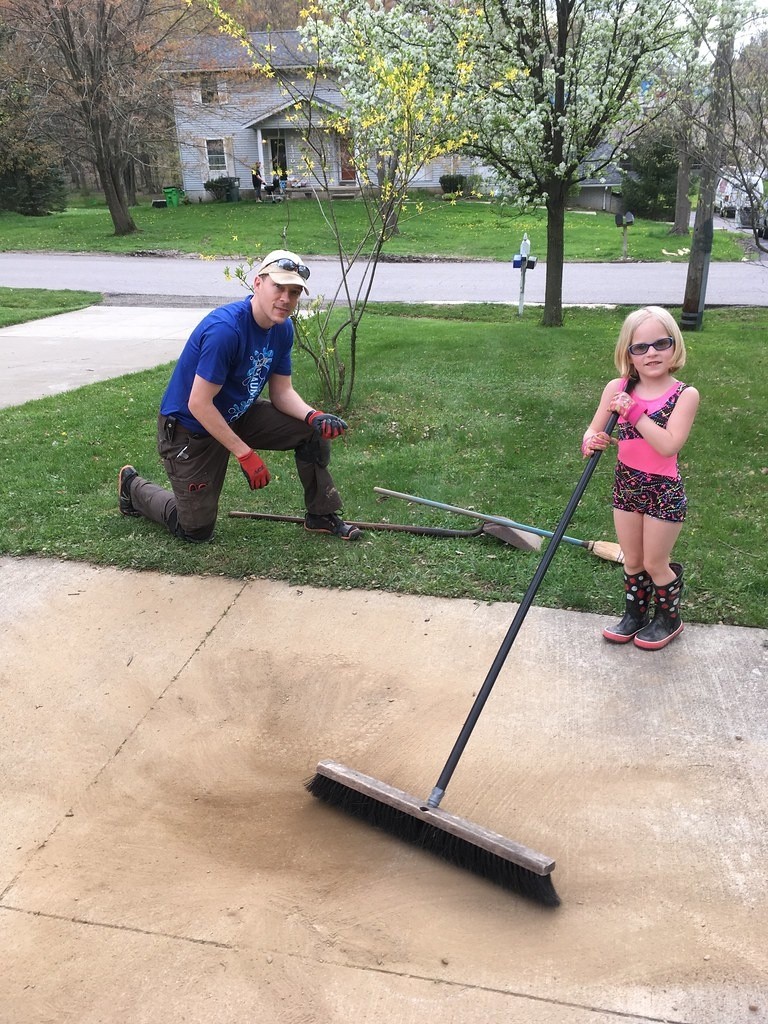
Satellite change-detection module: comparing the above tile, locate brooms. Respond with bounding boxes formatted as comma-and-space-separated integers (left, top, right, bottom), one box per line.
301, 407, 620, 905
373, 487, 626, 563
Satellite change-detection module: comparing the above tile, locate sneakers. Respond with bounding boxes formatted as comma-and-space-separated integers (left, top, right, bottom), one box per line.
304, 508, 360, 540
117, 465, 141, 519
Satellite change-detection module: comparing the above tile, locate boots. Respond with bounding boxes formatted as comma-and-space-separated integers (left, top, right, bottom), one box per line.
602, 566, 653, 644
633, 563, 685, 650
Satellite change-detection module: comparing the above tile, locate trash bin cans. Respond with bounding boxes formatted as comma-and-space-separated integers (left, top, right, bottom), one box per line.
162, 186, 179, 207
222, 176, 241, 202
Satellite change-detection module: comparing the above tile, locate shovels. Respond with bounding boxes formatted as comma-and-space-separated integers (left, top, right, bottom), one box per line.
228, 510, 544, 552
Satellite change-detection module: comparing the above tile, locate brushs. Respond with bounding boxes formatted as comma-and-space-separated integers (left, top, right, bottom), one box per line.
299, 374, 639, 913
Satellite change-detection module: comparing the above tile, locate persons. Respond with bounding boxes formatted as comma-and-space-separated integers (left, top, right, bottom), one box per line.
251, 161, 264, 203
118, 250, 360, 541
583, 306, 699, 649
279, 169, 287, 194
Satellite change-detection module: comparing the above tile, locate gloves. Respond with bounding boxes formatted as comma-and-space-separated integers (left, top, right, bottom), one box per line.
581, 431, 618, 460
606, 391, 648, 428
236, 449, 271, 489
308, 412, 348, 439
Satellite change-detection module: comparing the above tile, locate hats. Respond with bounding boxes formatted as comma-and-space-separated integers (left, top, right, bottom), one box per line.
258, 250, 309, 296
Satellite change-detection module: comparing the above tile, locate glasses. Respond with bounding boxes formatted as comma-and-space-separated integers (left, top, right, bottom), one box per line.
259, 258, 311, 280
627, 338, 674, 355
